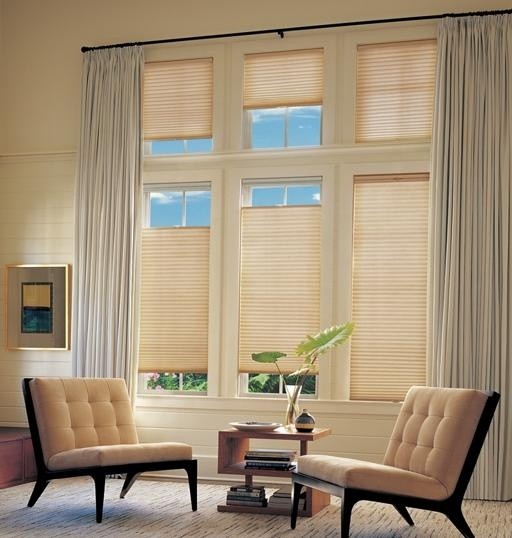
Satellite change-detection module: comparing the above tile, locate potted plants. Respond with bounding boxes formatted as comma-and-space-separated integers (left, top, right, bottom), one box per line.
248, 320, 357, 426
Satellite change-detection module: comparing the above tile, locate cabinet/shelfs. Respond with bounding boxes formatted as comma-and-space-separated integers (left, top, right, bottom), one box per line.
216, 426, 332, 517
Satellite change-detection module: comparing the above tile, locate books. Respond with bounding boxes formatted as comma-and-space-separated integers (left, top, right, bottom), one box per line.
225, 450, 306, 510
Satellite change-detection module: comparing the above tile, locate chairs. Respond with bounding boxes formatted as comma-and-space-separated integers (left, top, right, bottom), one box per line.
290, 385, 504, 537
21, 373, 197, 521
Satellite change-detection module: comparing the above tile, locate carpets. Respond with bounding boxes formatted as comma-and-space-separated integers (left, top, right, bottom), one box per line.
0, 472, 512, 537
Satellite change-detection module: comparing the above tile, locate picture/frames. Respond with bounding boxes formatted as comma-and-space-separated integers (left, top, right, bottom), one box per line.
3, 263, 72, 352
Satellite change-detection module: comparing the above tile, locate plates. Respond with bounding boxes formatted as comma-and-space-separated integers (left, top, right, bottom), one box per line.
229, 421, 283, 432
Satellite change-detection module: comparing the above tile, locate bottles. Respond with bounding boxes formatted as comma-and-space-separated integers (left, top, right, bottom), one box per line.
295, 408, 316, 433
